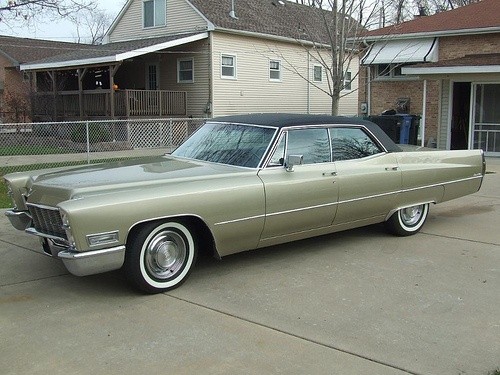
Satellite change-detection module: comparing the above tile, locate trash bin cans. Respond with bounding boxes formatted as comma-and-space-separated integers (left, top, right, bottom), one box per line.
373, 114, 404, 144
395, 112, 412, 145
408, 114, 422, 145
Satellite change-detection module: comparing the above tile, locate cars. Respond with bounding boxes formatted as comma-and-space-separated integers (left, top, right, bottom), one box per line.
3, 112, 487, 295
